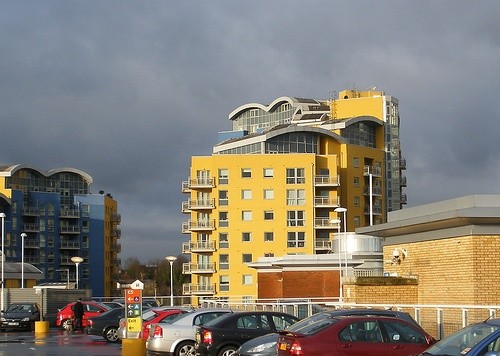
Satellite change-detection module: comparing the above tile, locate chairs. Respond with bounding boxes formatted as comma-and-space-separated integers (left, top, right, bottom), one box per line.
356, 330, 379, 342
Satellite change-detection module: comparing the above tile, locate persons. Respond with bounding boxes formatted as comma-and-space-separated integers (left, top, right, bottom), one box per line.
74, 298, 84, 332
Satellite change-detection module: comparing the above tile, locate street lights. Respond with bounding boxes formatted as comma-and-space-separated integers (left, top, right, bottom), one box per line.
333, 207, 348, 281
0, 213, 6, 316
20, 233, 28, 289
165, 256, 177, 307
328, 219, 343, 303
71, 256, 84, 290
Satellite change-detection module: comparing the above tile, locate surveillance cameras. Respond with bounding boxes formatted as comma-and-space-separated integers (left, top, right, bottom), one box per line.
393, 248, 399, 258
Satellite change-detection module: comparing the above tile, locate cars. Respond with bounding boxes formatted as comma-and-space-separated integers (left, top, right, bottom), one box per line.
0, 301, 40, 333
145, 308, 234, 356
416, 316, 500, 356
56, 298, 194, 342
194, 310, 301, 356
232, 307, 420, 356
275, 315, 441, 356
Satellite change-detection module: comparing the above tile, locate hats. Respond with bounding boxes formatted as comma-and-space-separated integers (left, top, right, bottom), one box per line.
77, 297, 82, 300
